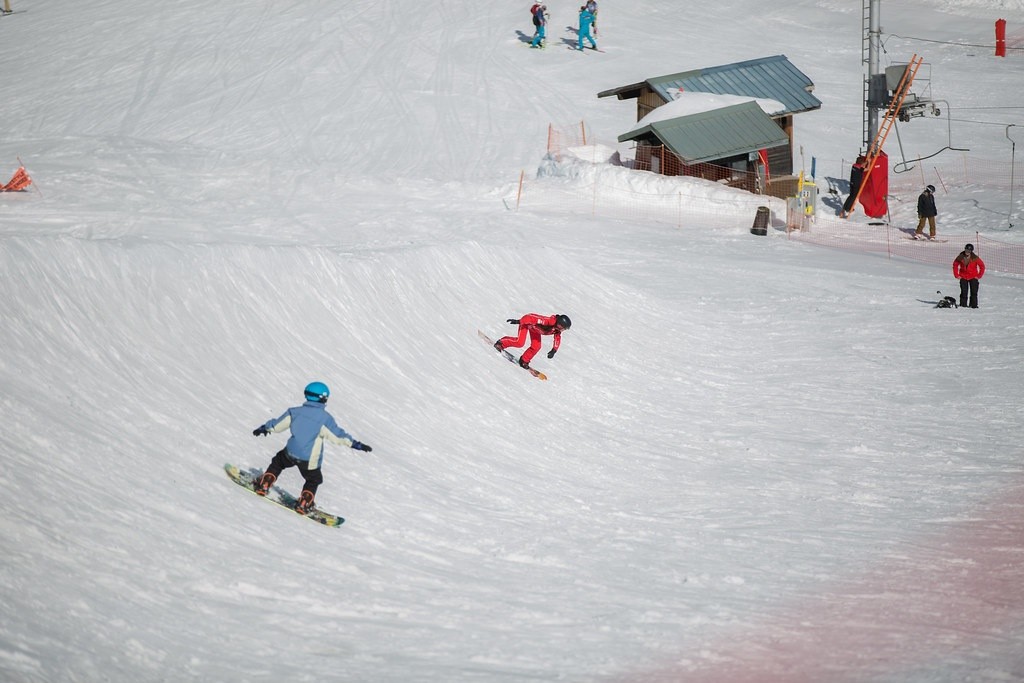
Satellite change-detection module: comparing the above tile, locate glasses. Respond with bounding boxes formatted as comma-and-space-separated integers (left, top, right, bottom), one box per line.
304, 390, 328, 403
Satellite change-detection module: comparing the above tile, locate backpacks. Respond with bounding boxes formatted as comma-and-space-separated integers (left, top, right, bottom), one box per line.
531, 4, 541, 14
533, 10, 544, 26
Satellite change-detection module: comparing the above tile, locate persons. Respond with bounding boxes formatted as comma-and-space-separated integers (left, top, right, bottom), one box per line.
952, 243, 985, 308
493, 313, 572, 370
529, 0, 551, 48
843, 156, 871, 212
251, 380, 373, 514
577, 0, 600, 51
916, 184, 938, 241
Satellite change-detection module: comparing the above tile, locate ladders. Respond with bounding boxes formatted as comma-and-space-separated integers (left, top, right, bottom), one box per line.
838, 53, 924, 220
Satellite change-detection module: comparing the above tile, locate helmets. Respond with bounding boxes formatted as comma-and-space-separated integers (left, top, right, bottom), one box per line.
926, 185, 935, 193
556, 314, 571, 329
965, 244, 973, 250
305, 382, 330, 402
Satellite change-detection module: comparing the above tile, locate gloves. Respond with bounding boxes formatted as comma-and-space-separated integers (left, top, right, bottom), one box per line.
350, 440, 372, 452
507, 319, 520, 324
548, 349, 556, 358
253, 425, 268, 437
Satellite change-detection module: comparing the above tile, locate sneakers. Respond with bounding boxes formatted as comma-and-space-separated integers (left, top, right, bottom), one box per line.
254, 473, 276, 496
295, 490, 315, 515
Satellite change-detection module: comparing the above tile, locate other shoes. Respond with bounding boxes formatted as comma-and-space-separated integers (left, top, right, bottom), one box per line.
960, 302, 967, 307
494, 340, 504, 353
916, 234, 921, 238
969, 304, 978, 308
930, 236, 935, 241
845, 210, 849, 217
519, 356, 529, 369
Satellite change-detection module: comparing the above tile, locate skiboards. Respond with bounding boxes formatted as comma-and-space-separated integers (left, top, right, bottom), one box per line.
567, 46, 589, 55
573, 42, 605, 53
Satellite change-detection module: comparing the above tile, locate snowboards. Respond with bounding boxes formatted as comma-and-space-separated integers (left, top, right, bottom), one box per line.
901, 237, 948, 243
477, 329, 547, 380
224, 462, 344, 527
